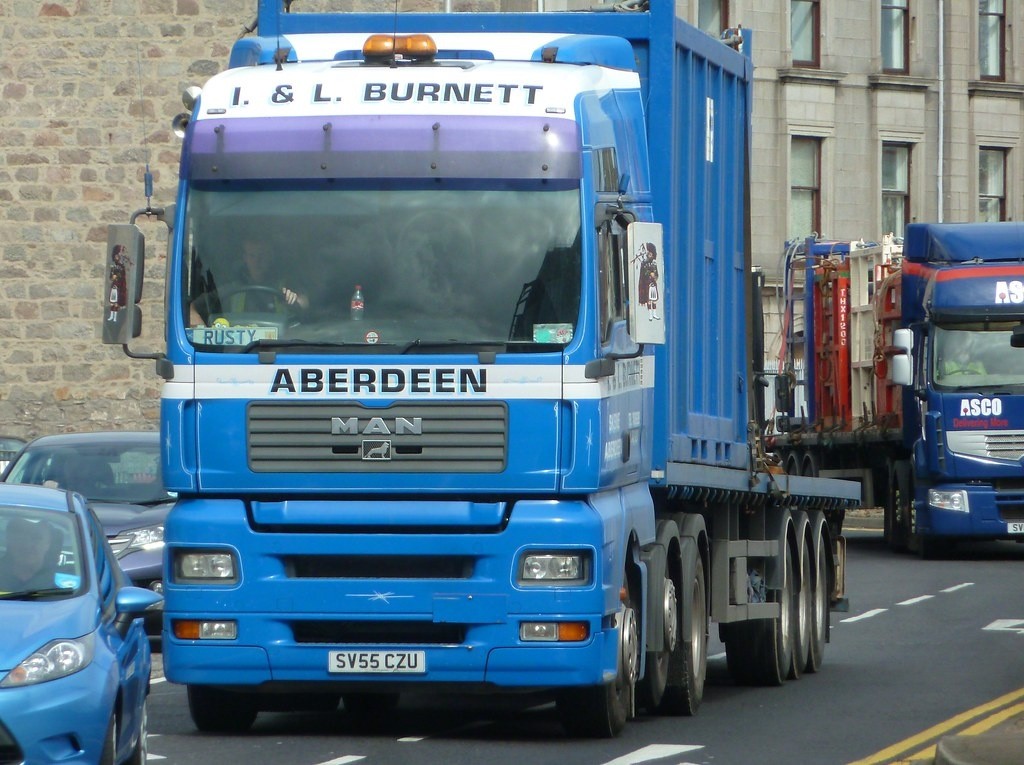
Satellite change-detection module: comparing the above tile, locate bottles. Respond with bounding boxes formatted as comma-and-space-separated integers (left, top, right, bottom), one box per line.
352, 284, 364, 320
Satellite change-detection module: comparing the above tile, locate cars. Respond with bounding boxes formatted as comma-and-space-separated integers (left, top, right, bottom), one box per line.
2, 430, 171, 649
0, 479, 166, 765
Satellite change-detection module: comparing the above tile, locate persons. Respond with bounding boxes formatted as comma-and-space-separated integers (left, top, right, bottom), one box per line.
190, 232, 309, 328
55, 459, 96, 491
0, 524, 67, 592
937, 345, 987, 380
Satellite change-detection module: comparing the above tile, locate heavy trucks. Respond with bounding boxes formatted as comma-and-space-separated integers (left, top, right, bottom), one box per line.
752, 224, 1024, 560
100, 0, 865, 740
